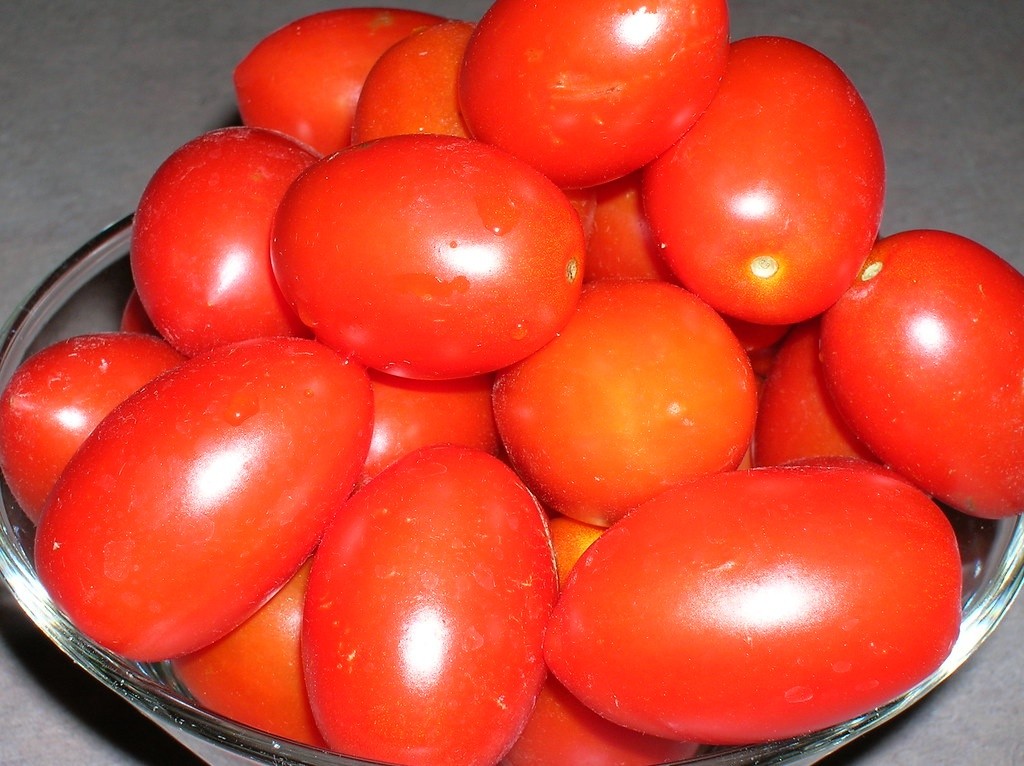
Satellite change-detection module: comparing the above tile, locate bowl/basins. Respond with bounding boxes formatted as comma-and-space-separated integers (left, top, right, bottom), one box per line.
1, 183, 1024, 766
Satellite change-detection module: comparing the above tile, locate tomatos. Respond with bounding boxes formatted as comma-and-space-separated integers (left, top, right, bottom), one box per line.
2, 0, 1023, 765
